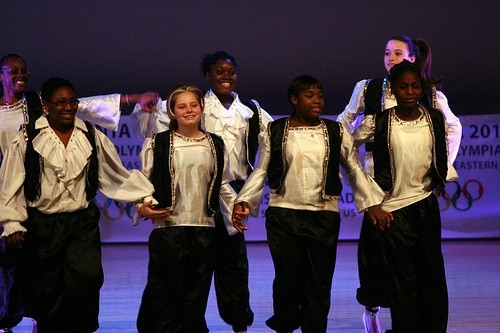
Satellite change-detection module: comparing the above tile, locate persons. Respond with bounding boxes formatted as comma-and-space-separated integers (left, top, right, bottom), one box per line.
351, 59, 449, 333
232, 74, 394, 333
132, 86, 249, 333
337, 32, 462, 333
150, 50, 274, 333
0, 76, 160, 333
0, 53, 159, 333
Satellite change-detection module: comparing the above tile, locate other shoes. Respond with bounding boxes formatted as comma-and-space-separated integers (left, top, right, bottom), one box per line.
363, 310, 383, 333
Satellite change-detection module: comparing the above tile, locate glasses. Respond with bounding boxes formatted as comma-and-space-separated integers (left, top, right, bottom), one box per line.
3, 65, 30, 75
46, 98, 80, 108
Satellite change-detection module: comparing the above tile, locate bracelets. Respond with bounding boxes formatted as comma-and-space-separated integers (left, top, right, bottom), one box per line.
125, 93, 132, 107
